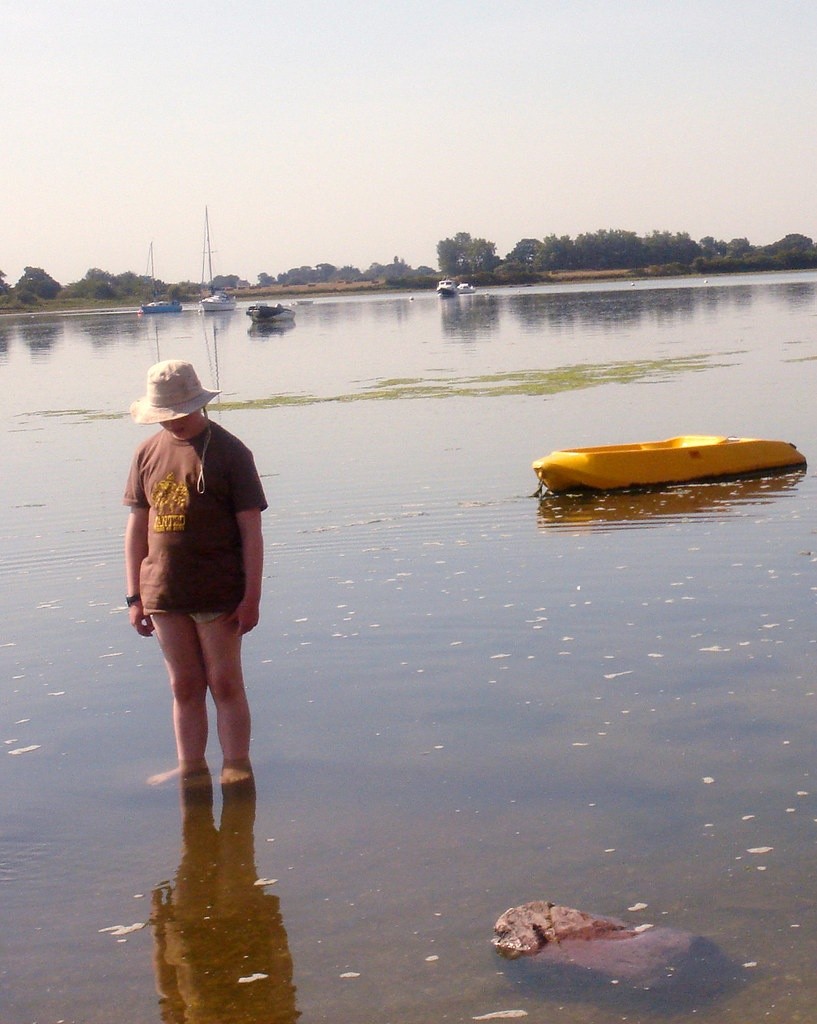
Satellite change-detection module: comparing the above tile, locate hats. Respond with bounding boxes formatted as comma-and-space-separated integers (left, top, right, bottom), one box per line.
130, 359, 221, 424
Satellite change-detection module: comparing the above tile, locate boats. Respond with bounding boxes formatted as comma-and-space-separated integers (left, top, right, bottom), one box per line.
532, 435, 809, 496
245, 301, 295, 323
458, 284, 476, 296
437, 275, 458, 297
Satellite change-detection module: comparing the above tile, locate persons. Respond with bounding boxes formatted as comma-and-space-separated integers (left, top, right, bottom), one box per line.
121, 360, 268, 762
148, 772, 303, 1024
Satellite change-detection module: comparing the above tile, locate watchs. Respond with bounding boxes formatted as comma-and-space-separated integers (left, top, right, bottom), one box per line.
126, 593, 140, 606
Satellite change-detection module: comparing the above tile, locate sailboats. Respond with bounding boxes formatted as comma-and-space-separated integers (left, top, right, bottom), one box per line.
143, 241, 183, 313
203, 204, 237, 311
